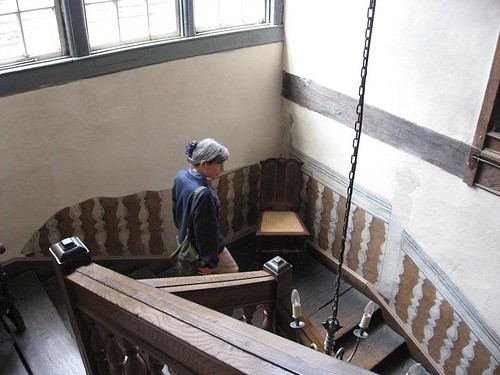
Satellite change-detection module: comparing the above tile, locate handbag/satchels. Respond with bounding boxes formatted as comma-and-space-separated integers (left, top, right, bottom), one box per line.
170, 242, 201, 276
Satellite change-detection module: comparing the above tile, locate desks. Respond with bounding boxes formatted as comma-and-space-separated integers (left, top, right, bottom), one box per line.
255, 211, 310, 271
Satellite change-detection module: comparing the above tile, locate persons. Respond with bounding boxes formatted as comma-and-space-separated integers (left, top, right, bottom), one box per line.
172, 138, 239, 275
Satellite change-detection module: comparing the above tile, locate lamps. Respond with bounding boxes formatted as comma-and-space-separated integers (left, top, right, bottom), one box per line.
286, 0, 374, 365
